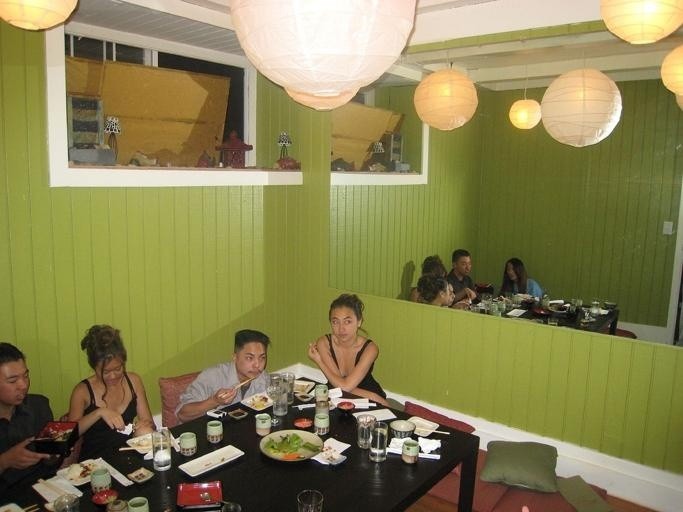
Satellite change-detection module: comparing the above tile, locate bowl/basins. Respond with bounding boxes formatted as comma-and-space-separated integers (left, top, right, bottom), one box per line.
603, 301, 616, 311
388, 419, 415, 438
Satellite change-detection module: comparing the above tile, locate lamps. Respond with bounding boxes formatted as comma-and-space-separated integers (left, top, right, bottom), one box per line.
600, 1, 683, 44
230, 1, 416, 98
1, 1, 78, 30
661, 45, 683, 95
541, 48, 623, 148
675, 96, 683, 110
284, 89, 359, 112
509, 78, 541, 129
413, 62, 478, 132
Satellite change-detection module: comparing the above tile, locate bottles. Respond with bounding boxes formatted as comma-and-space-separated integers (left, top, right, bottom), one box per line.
590, 298, 599, 318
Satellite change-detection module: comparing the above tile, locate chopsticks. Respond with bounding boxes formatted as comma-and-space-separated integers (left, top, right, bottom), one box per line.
217, 376, 258, 397
20, 504, 41, 511
35, 477, 67, 496
416, 427, 449, 435
118, 446, 150, 451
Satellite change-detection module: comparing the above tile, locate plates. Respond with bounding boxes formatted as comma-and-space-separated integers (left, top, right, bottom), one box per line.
56, 458, 101, 488
227, 408, 248, 421
515, 294, 532, 302
177, 444, 246, 479
533, 306, 550, 316
126, 466, 155, 484
0, 502, 24, 512
239, 391, 274, 412
257, 430, 323, 462
546, 303, 566, 315
322, 450, 347, 465
125, 431, 156, 454
174, 480, 221, 507
89, 490, 118, 505
407, 415, 439, 437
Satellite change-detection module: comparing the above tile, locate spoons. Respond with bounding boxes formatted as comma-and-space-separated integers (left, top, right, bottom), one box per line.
199, 493, 229, 506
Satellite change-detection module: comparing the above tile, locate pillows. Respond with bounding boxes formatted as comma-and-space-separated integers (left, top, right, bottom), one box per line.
158, 372, 202, 430
479, 441, 557, 492
403, 401, 475, 476
428, 449, 510, 512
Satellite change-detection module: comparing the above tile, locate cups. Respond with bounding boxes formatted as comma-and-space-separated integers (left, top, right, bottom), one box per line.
369, 420, 388, 463
206, 420, 223, 444
355, 413, 376, 448
127, 496, 149, 512
106, 499, 127, 512
547, 318, 558, 326
254, 412, 271, 436
89, 467, 112, 494
297, 489, 323, 512
151, 429, 172, 472
401, 439, 419, 464
572, 297, 583, 312
265, 371, 355, 435
469, 293, 521, 317
579, 318, 589, 328
52, 495, 79, 512
176, 432, 196, 456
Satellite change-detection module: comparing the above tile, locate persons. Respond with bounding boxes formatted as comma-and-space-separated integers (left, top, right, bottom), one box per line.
174, 329, 273, 423
409, 249, 482, 310
307, 293, 390, 407
0, 342, 55, 497
66, 324, 156, 464
498, 257, 543, 300
